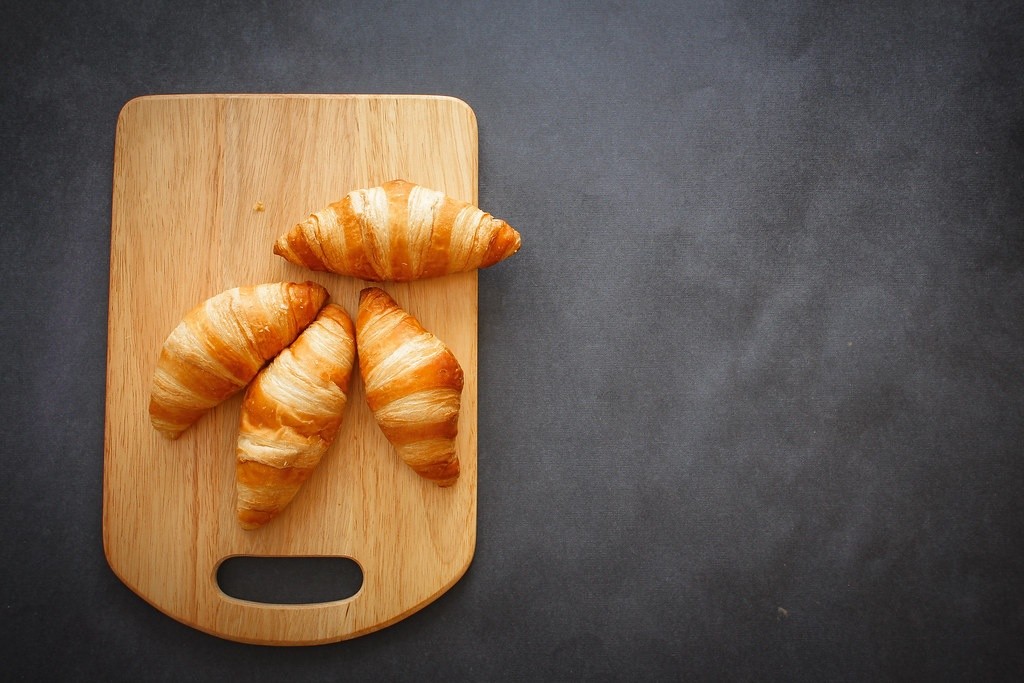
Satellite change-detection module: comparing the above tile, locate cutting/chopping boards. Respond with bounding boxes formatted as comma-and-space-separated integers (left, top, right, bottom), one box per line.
102, 93, 478, 647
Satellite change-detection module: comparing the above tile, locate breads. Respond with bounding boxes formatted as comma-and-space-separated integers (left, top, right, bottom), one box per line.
355, 288, 464, 488
148, 281, 328, 438
272, 180, 520, 282
236, 305, 356, 530
